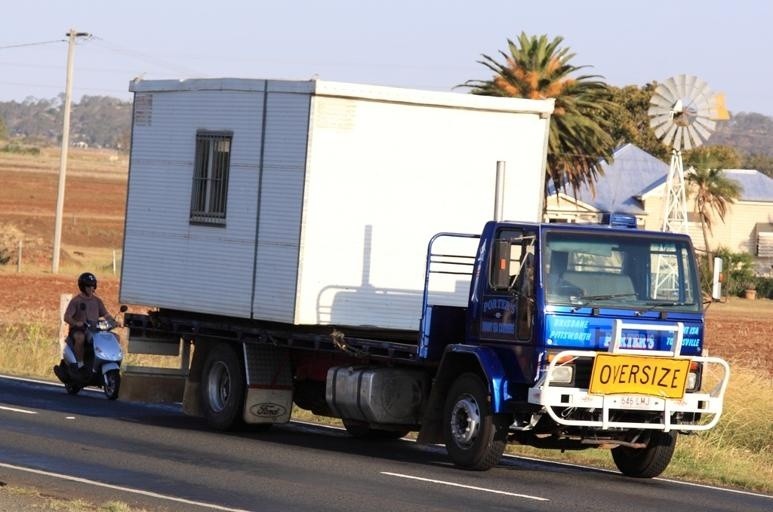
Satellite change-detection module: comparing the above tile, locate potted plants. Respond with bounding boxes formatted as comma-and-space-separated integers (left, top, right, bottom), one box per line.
744, 281, 756, 299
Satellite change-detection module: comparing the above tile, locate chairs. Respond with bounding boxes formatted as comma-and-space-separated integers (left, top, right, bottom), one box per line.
558, 271, 637, 302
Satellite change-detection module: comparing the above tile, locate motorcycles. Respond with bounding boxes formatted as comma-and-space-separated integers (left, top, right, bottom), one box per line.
54, 302, 128, 401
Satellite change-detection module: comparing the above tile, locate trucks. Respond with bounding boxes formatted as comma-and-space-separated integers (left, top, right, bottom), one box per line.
119, 77, 730, 478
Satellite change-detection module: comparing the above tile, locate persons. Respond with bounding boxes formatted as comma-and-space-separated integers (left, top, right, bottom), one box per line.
62, 271, 121, 376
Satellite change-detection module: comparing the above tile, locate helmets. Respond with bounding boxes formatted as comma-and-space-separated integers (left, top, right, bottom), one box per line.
78, 273, 96, 297
